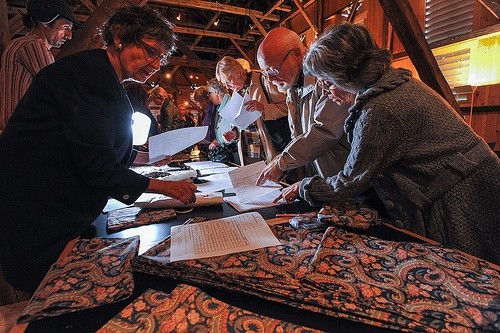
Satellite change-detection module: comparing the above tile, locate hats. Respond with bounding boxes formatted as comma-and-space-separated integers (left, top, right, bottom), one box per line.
26, 0, 86, 28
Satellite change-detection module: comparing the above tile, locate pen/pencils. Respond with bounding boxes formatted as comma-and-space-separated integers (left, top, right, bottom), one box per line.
275, 212, 317, 217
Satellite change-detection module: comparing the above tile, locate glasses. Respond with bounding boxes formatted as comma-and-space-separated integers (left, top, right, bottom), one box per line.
261, 48, 295, 77
318, 78, 336, 91
136, 35, 169, 66
207, 92, 212, 97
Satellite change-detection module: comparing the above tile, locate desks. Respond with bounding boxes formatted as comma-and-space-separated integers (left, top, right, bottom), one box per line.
24, 147, 499, 333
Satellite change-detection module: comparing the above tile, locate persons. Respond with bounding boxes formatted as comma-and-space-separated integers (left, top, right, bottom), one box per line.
271, 24, 500, 263
215, 56, 288, 164
0, 3, 197, 292
150, 78, 239, 148
243, 26, 352, 186
0, 0, 84, 131
